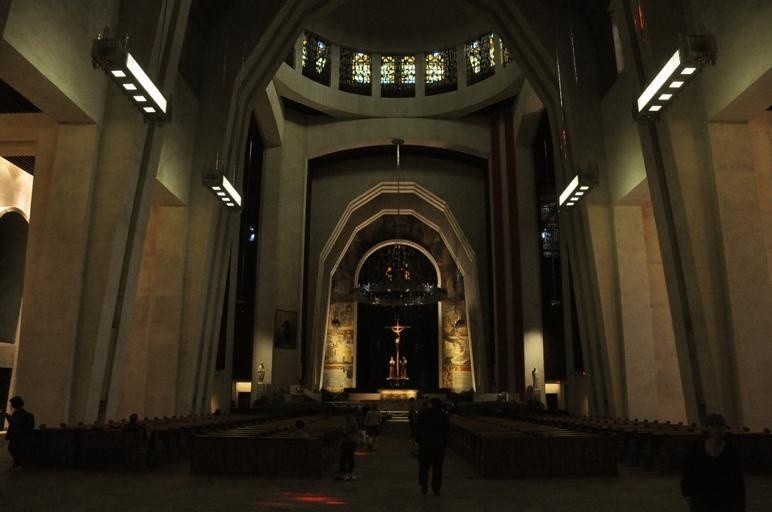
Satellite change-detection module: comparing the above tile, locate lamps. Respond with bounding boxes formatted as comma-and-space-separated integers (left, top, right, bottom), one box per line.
555, 173, 595, 212
97, 26, 168, 123
206, 172, 243, 211
633, 30, 712, 125
330, 138, 449, 307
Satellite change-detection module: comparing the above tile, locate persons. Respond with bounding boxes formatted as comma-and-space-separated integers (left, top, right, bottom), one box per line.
279, 313, 295, 344
121, 412, 143, 432
1, 395, 28, 472
286, 419, 313, 438
359, 403, 383, 451
334, 413, 359, 482
680, 412, 745, 511
406, 395, 463, 458
414, 396, 449, 497
